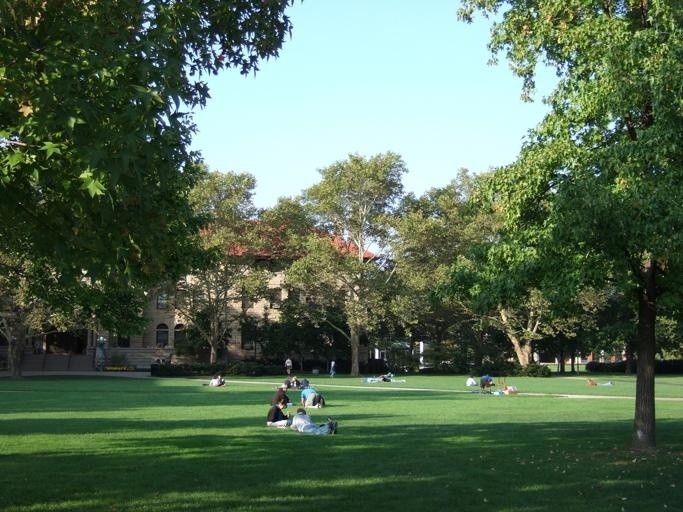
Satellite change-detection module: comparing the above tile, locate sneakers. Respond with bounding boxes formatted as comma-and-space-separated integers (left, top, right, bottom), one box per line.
331, 422, 337, 434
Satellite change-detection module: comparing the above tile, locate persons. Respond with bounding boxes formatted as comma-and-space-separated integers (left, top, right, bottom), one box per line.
265, 376, 337, 436
208, 374, 226, 387
283, 356, 292, 375
587, 378, 613, 385
361, 372, 394, 384
328, 359, 337, 377
465, 373, 517, 395
